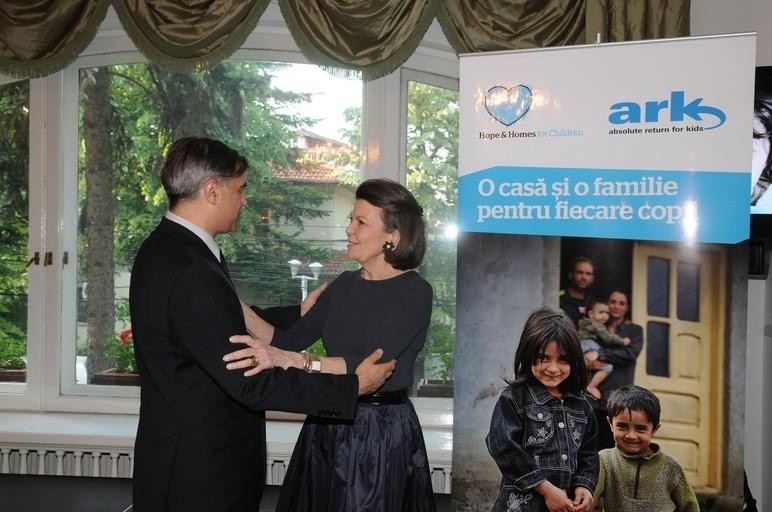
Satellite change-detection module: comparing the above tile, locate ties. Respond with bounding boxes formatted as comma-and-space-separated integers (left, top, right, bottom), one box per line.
219, 250, 232, 285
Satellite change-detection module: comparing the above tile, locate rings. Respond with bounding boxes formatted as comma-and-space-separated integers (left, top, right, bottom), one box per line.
249, 357, 259, 368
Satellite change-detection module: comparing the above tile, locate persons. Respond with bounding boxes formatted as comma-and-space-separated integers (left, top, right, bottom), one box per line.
129, 138, 399, 510
750, 93, 771, 216
485, 310, 600, 512
560, 254, 596, 324
580, 286, 644, 448
591, 385, 701, 512
579, 300, 630, 401
222, 179, 438, 510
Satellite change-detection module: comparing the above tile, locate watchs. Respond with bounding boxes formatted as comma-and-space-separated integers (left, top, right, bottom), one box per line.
309, 354, 321, 374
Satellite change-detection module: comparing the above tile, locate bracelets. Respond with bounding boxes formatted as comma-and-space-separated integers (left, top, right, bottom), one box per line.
302, 352, 310, 374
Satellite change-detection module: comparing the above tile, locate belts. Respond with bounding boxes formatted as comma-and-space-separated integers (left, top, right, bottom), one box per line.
359, 391, 409, 407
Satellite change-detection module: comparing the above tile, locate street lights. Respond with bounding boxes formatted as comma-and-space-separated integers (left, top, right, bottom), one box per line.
286, 258, 323, 304
